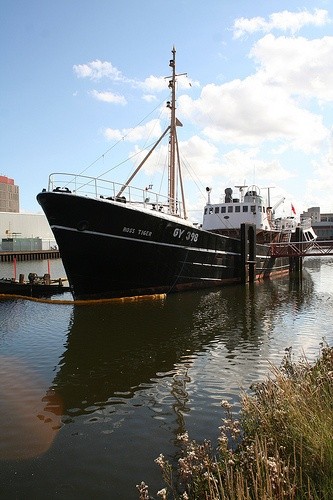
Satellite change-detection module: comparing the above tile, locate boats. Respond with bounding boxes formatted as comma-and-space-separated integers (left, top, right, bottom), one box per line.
297, 207, 332, 243
34, 46, 317, 301
